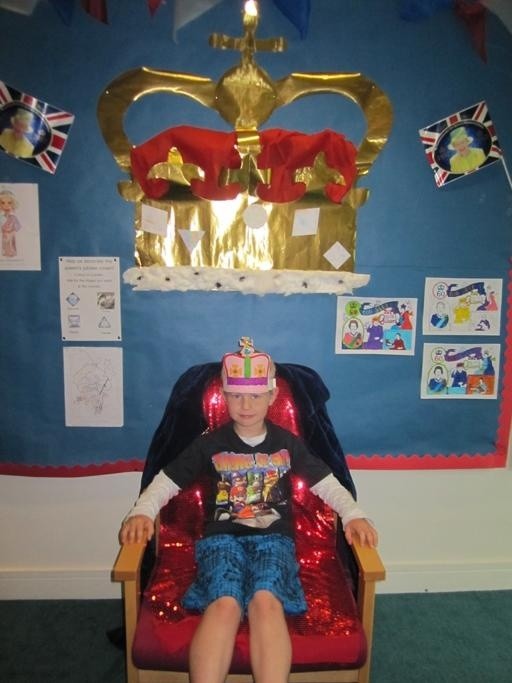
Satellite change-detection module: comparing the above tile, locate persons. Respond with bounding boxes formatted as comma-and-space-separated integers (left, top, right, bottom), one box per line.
341, 281, 497, 395
1, 109, 36, 156
447, 126, 485, 173
1, 189, 22, 256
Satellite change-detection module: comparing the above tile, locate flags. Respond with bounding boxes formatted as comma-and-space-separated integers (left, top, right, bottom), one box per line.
0, 80, 76, 174
417, 99, 503, 188
